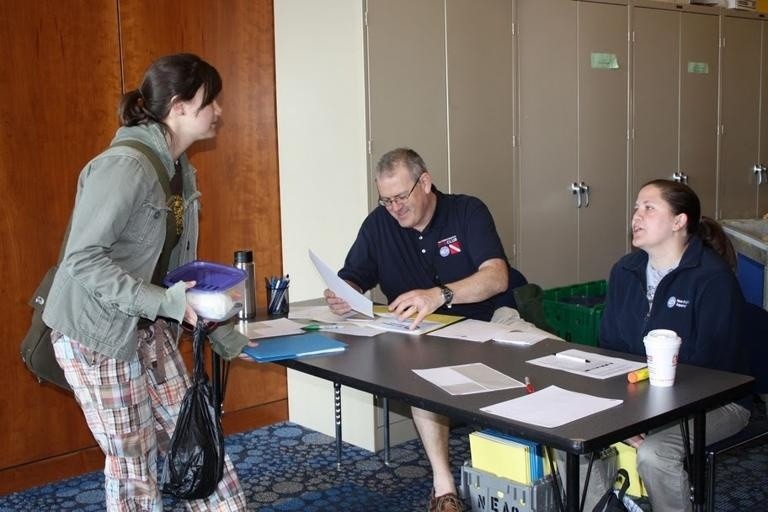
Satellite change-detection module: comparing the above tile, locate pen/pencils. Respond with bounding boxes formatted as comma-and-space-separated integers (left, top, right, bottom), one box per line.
302, 325, 340, 329
551, 352, 589, 364
524, 376, 534, 394
264, 274, 290, 314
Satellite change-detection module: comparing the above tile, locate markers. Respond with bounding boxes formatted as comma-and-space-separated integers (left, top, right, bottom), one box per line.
627, 367, 649, 384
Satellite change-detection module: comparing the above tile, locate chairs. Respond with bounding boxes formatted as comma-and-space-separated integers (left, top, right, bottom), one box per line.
685, 302, 768, 512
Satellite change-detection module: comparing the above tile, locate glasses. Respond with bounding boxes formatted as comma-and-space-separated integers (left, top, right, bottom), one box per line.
378, 178, 421, 207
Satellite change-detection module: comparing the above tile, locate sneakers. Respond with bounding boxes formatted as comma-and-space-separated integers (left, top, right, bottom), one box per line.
427, 488, 461, 512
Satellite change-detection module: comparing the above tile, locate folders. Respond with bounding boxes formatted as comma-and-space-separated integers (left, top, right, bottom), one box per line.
243, 332, 352, 363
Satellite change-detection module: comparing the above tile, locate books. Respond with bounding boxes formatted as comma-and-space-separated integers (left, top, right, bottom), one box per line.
236, 306, 649, 430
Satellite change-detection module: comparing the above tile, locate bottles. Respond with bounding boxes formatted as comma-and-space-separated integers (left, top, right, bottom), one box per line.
232, 250, 257, 319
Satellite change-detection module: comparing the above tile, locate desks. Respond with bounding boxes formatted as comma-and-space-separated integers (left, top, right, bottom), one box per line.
181, 295, 759, 512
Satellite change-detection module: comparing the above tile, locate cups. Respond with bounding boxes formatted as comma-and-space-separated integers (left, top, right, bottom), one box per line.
643, 329, 683, 388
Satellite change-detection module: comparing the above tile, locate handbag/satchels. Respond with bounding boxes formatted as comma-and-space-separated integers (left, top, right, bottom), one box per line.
20, 263, 74, 390
592, 469, 652, 512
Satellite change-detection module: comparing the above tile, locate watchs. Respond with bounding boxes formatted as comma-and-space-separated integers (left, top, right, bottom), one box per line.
436, 282, 454, 306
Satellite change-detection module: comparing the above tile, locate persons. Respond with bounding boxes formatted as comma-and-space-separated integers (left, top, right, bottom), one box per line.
552, 180, 762, 512
42, 53, 248, 512
323, 149, 529, 511
